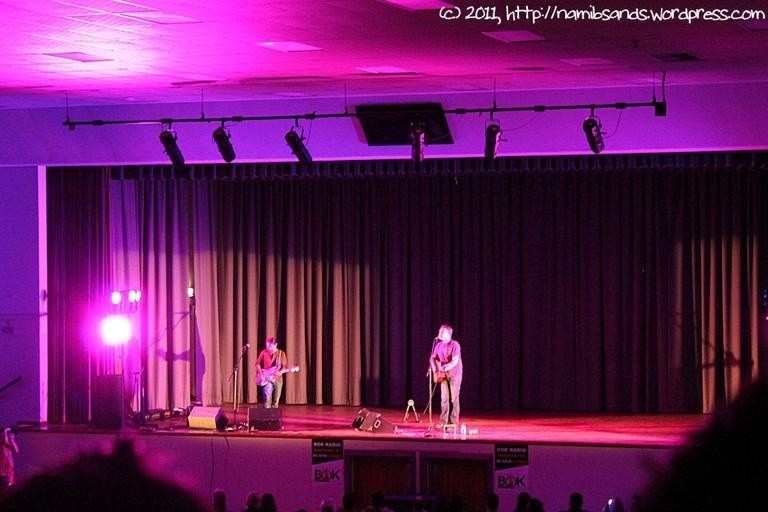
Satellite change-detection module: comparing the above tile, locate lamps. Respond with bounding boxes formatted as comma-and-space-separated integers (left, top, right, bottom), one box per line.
156, 106, 606, 169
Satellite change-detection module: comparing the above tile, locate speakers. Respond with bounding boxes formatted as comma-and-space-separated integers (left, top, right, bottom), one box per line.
187, 405, 228, 430
352, 408, 393, 433
249, 407, 284, 431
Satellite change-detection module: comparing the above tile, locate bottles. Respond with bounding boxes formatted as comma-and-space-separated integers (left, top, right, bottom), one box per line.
460, 423, 466, 440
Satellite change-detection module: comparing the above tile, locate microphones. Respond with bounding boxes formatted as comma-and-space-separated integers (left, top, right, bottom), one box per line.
435, 337, 438, 343
241, 344, 250, 353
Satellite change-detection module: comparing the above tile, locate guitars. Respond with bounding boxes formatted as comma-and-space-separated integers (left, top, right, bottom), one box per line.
432, 358, 448, 384
256, 366, 300, 386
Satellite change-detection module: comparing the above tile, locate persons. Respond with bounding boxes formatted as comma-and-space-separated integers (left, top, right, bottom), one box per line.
0, 428, 20, 486
429, 324, 464, 431
210, 486, 644, 512
254, 336, 289, 409
